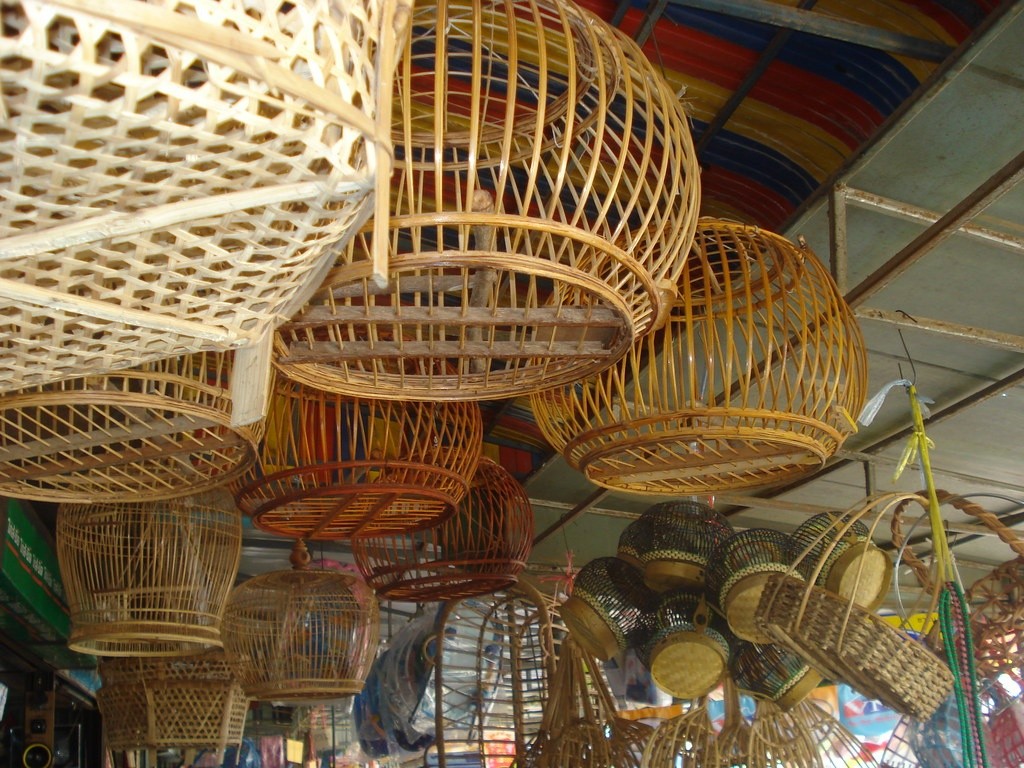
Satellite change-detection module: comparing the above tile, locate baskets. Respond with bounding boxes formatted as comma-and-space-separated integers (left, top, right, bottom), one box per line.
1, 350, 1024, 768
1, 1, 411, 397
528, 217, 866, 498
270, 0, 701, 403
